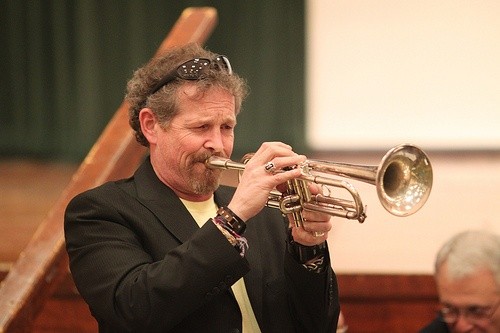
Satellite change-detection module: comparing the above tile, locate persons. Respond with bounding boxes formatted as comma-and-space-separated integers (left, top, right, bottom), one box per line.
419, 228, 500, 333
64, 42, 342, 333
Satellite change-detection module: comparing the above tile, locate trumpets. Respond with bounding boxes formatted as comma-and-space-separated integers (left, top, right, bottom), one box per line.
205, 143, 434, 237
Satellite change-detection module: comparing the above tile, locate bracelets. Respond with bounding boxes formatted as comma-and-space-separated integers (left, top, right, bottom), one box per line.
289, 241, 328, 270
215, 205, 247, 237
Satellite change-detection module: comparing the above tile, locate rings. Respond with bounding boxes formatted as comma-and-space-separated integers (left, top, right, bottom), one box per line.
263, 163, 276, 172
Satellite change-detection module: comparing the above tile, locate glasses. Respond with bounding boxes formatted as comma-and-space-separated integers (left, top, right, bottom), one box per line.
434, 299, 500, 324
137, 55, 233, 106
